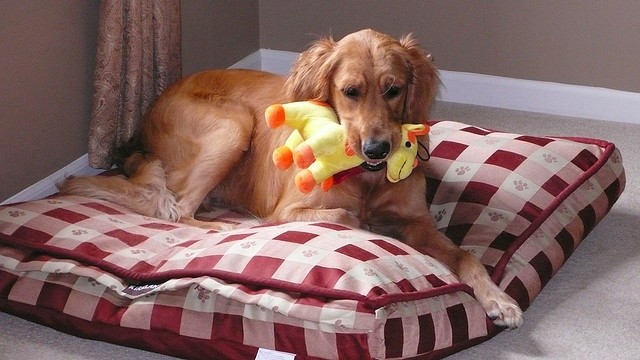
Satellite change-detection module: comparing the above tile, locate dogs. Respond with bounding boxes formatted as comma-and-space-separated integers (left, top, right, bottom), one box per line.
55, 28, 525, 330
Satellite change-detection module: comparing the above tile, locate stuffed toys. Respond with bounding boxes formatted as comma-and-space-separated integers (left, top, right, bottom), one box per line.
265, 101, 430, 194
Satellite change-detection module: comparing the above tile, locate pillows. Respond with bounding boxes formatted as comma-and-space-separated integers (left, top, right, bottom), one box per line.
1, 120, 626, 360
